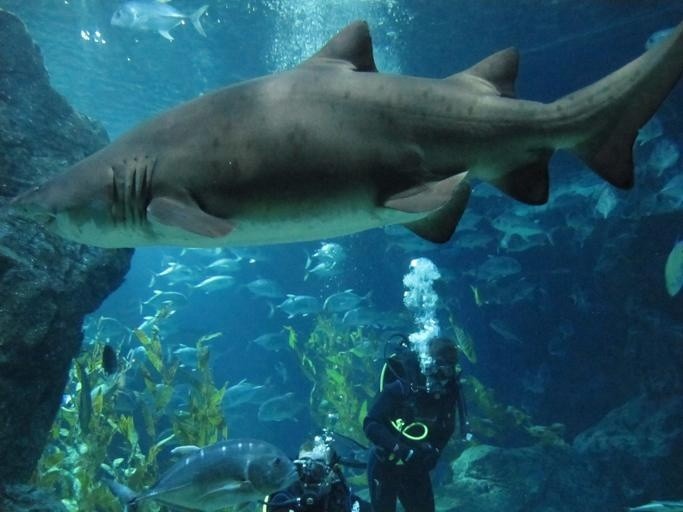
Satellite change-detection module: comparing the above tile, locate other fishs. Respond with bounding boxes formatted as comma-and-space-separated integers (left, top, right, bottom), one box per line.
0, 2, 683, 512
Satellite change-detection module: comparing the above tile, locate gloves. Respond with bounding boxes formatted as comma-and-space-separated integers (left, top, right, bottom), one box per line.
392, 446, 441, 472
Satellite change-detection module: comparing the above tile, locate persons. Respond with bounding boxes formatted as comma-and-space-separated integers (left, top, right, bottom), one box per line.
363, 337, 460, 512
266, 436, 371, 512
